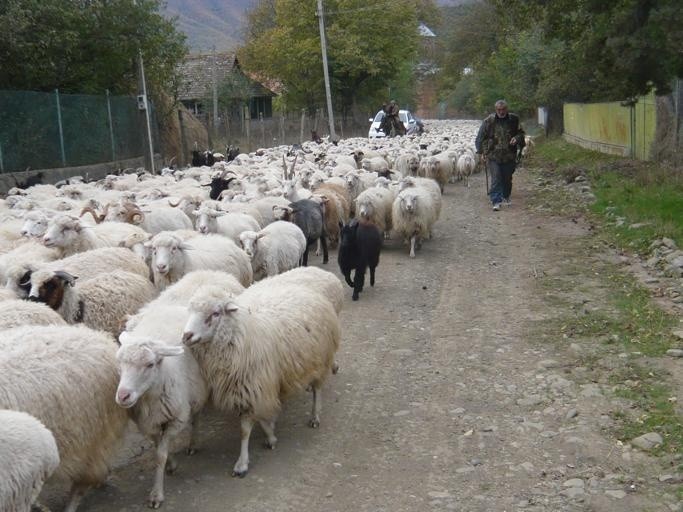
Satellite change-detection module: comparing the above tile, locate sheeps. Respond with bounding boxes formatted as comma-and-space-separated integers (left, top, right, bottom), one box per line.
1, 114, 535, 512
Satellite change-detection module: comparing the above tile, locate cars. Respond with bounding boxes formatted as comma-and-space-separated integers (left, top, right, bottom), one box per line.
365, 108, 418, 139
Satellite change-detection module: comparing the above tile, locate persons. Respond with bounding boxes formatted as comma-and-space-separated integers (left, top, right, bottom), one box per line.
375, 100, 399, 132
475, 100, 524, 211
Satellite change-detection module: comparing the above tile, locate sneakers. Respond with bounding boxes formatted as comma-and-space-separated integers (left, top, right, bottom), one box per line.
504, 196, 511, 206
493, 202, 501, 210
